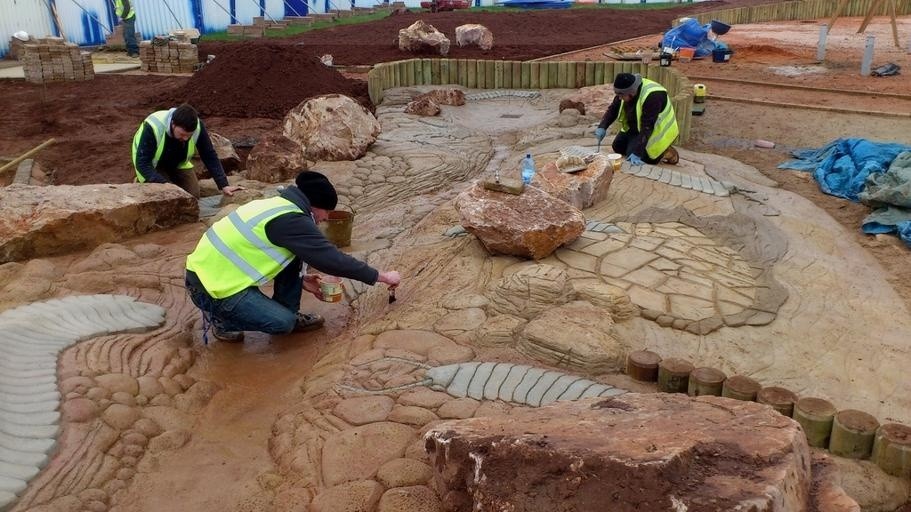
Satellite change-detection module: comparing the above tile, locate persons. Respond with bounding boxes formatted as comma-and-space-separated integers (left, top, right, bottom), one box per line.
112, 0, 139, 58
186, 171, 401, 343
594, 71, 680, 165
131, 103, 248, 200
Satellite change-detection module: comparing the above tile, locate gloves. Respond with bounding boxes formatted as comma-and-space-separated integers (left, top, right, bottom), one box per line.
594, 127, 605, 141
625, 153, 644, 166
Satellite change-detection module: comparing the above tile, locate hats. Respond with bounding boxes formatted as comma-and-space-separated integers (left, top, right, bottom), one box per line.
612, 73, 641, 95
295, 171, 337, 210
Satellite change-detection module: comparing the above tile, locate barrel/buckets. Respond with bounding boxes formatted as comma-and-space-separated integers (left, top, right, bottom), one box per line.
519, 154, 537, 181
694, 83, 706, 104
679, 47, 696, 62
321, 202, 356, 249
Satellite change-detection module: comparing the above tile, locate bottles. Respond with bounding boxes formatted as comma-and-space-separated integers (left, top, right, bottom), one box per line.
521, 154, 536, 185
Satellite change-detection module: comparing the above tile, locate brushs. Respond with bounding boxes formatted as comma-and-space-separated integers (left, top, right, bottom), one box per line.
389, 288, 396, 303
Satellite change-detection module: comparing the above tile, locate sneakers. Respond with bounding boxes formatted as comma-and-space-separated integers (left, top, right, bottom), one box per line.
209, 313, 244, 342
293, 311, 325, 333
663, 145, 678, 164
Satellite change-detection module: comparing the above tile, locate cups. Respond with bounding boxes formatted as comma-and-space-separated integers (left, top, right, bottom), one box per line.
321, 274, 344, 302
608, 153, 622, 171
